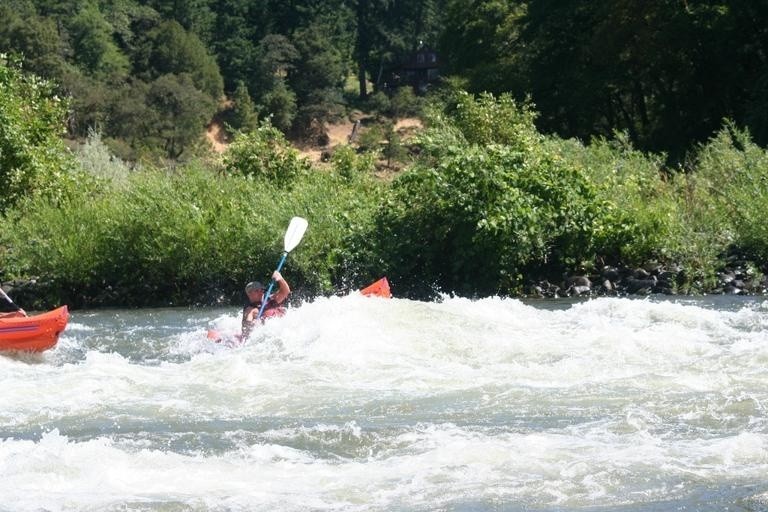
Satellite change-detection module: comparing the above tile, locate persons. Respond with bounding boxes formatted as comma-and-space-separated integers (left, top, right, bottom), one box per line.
240, 270, 291, 341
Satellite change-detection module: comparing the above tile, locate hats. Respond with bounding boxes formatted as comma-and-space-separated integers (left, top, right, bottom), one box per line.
245, 281, 265, 294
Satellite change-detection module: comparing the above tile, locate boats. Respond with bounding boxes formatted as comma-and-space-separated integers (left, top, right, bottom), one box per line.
0, 304, 69, 354
206, 277, 390, 349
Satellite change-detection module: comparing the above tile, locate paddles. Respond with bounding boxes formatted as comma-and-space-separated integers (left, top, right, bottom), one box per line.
255, 217, 308, 318
